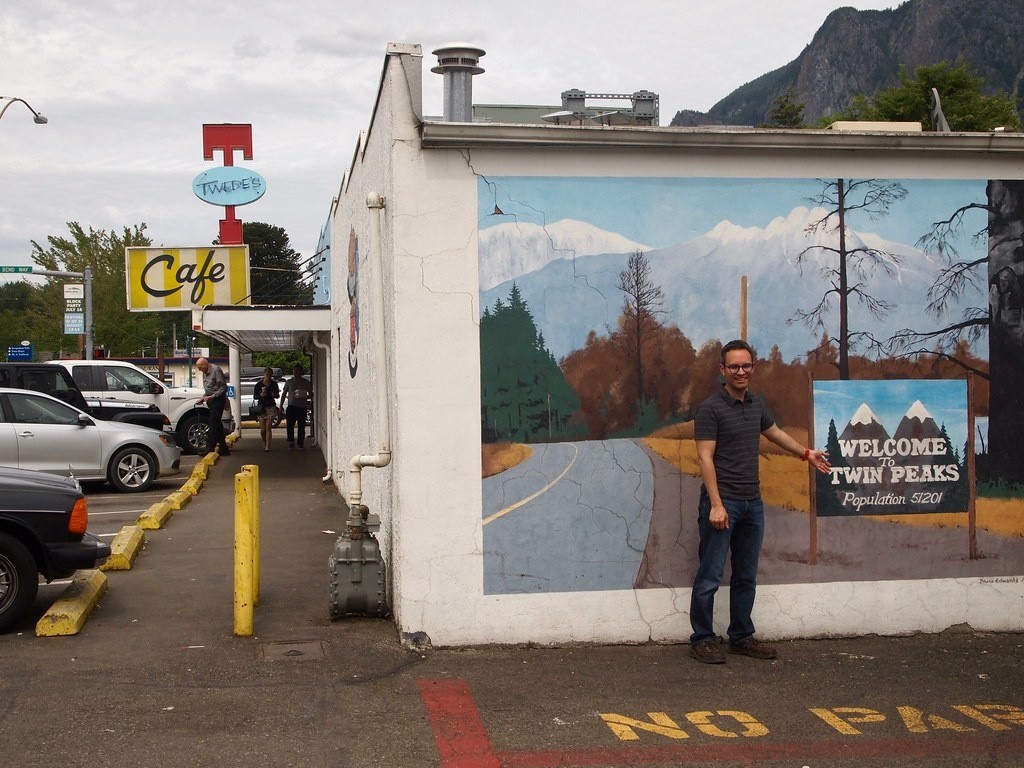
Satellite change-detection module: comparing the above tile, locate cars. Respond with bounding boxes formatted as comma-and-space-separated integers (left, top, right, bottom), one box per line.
0, 460, 111, 632
0, 387, 181, 493
223, 381, 313, 431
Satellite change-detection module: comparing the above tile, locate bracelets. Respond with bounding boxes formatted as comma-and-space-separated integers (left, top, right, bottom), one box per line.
802, 450, 809, 461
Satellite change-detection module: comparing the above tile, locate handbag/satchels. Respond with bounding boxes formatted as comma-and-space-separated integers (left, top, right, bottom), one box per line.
249, 399, 266, 416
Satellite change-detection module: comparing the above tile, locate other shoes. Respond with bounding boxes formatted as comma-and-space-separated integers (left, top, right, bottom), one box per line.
264, 447, 271, 452
199, 450, 215, 456
217, 449, 231, 456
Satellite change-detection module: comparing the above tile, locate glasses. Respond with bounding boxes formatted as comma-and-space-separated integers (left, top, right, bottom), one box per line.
725, 363, 755, 374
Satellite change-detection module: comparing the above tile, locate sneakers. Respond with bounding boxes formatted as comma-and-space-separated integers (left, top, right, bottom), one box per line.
298, 446, 305, 450
288, 443, 296, 450
690, 640, 727, 663
729, 638, 778, 659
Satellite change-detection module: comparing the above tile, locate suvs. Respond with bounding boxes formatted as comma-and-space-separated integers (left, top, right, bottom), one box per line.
53, 359, 233, 454
1, 362, 93, 418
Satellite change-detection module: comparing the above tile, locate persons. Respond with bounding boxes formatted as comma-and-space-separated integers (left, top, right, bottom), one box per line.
278, 364, 312, 451
196, 358, 230, 456
690, 340, 832, 664
254, 367, 279, 452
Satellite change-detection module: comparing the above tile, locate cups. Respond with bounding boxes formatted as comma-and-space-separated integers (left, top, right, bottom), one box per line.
262, 387, 266, 392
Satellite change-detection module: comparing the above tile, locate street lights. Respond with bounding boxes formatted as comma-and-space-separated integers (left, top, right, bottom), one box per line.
0, 97, 49, 125
